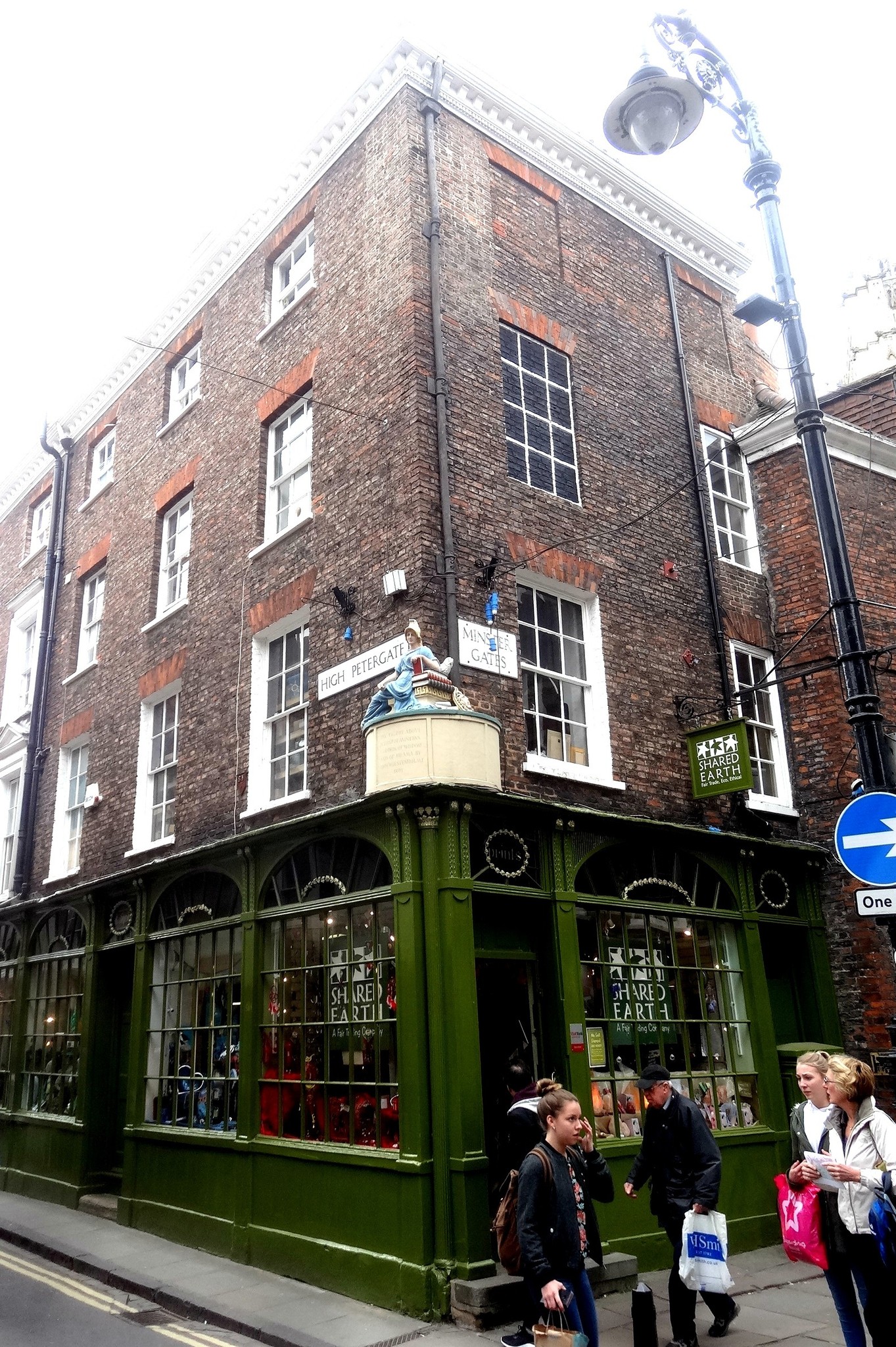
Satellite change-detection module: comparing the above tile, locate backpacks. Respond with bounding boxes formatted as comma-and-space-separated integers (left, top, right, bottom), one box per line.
490, 1148, 553, 1275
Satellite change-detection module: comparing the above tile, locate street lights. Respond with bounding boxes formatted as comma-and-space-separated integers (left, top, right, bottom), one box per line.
597, 13, 896, 791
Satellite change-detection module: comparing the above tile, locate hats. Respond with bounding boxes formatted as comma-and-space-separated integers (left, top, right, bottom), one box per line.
634, 1064, 672, 1089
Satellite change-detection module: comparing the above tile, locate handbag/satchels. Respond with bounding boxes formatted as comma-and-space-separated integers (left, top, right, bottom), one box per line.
869, 1171, 896, 1267
679, 1208, 736, 1294
532, 1308, 589, 1347
774, 1173, 829, 1269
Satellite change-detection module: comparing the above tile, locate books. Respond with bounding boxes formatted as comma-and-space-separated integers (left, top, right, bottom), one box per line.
411, 657, 455, 694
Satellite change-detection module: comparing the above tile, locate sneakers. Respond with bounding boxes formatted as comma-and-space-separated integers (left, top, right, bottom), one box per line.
500, 1324, 534, 1347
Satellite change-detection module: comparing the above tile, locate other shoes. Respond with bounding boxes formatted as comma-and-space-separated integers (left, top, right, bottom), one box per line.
708, 1303, 741, 1337
665, 1337, 700, 1347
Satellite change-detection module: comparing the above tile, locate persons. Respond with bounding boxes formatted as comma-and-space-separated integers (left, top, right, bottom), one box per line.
516, 1078, 613, 1347
802, 1053, 896, 1347
499, 1057, 555, 1347
620, 1064, 741, 1347
786, 1051, 870, 1347
359, 620, 441, 729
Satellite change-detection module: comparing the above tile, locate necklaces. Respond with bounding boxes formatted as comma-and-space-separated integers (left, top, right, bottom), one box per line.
847, 1123, 853, 1130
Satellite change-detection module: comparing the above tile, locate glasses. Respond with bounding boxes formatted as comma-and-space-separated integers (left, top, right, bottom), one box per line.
823, 1077, 841, 1084
643, 1083, 664, 1092
